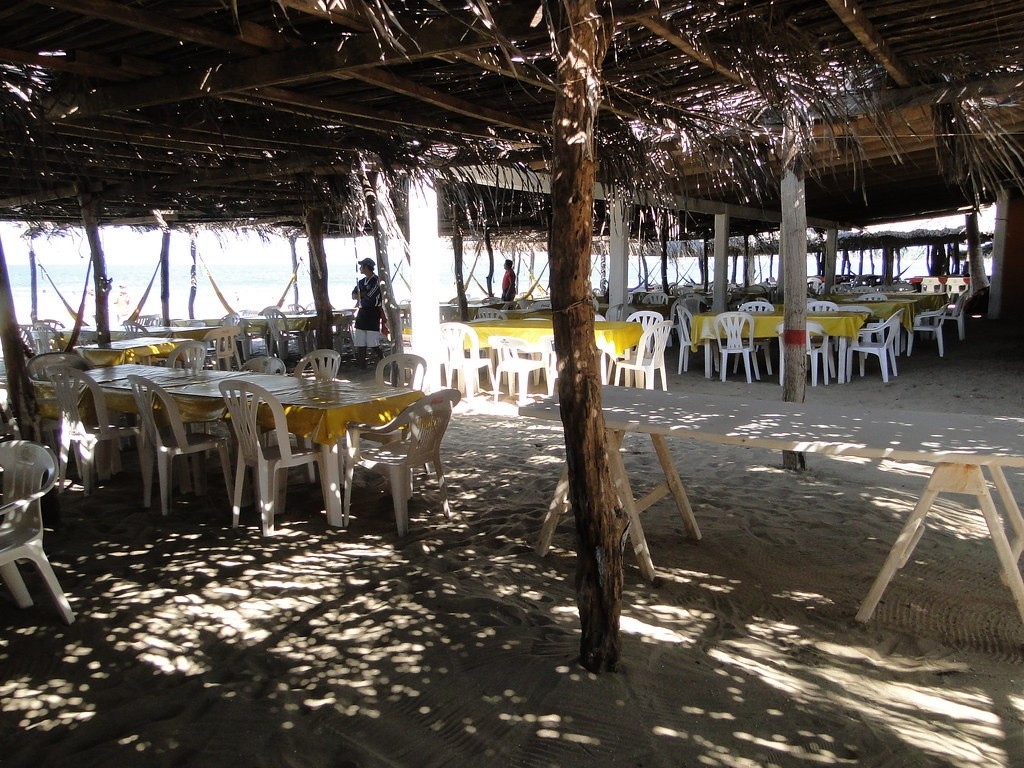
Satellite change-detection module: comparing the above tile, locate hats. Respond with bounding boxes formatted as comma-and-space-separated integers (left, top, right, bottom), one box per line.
358, 258, 376, 267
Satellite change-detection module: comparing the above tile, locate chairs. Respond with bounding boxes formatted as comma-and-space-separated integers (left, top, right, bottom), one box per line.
0, 264, 965, 626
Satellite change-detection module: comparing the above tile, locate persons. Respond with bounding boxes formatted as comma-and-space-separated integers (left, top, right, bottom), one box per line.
351, 258, 382, 368
502, 259, 516, 302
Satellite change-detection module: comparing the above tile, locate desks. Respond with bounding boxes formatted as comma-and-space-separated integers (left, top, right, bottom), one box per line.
687, 310, 870, 386
73, 334, 190, 364
831, 291, 948, 338
519, 386, 1024, 626
47, 365, 424, 530
81, 324, 219, 342
769, 297, 918, 356
401, 319, 642, 389
190, 315, 334, 332
